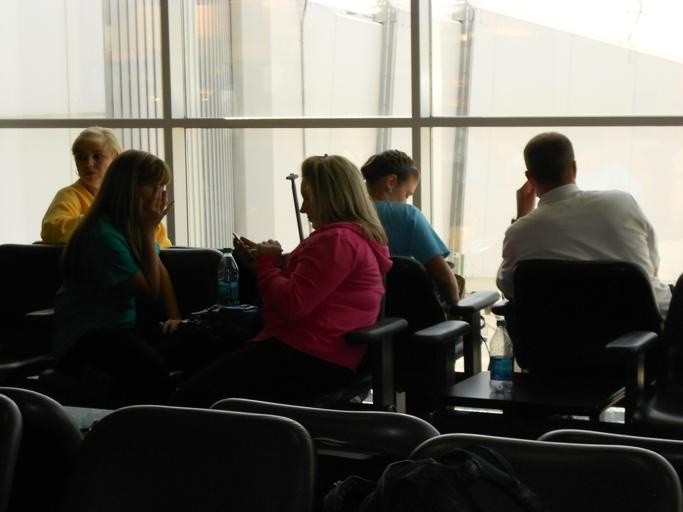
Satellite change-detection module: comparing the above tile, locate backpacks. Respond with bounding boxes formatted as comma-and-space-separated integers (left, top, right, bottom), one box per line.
324, 446, 548, 512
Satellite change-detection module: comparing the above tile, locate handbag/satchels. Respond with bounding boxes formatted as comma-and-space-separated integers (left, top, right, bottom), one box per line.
188, 304, 263, 338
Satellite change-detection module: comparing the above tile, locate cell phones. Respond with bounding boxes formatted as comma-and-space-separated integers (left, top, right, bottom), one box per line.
232, 231, 255, 259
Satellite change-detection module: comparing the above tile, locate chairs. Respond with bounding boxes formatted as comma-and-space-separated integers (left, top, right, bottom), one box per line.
0, 242, 683, 512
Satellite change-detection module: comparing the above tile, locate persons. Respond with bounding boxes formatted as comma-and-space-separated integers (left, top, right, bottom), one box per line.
494, 132, 673, 331
40, 125, 174, 248
360, 149, 466, 323
175, 154, 394, 409
49, 149, 182, 410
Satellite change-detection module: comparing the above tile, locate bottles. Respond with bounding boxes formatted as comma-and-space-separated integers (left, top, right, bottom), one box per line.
216, 247, 239, 303
488, 316, 515, 393
